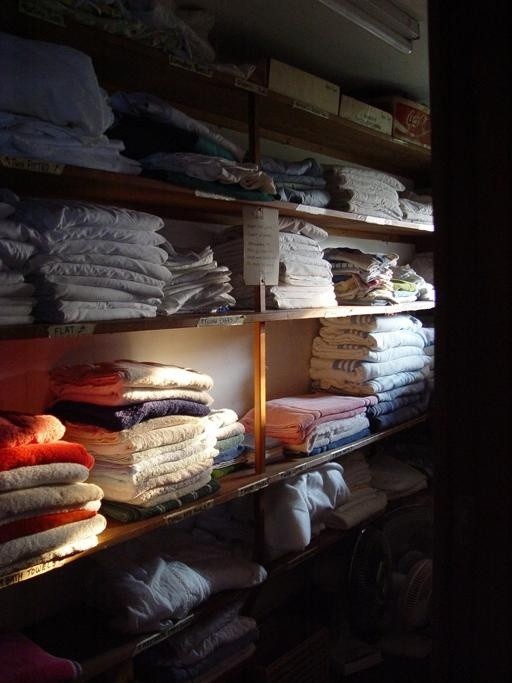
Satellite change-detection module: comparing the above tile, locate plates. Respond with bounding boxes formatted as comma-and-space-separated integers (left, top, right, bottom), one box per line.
1, 8, 439, 678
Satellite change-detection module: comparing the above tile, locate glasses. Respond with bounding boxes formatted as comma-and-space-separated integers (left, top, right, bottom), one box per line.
319, 0, 420, 57
